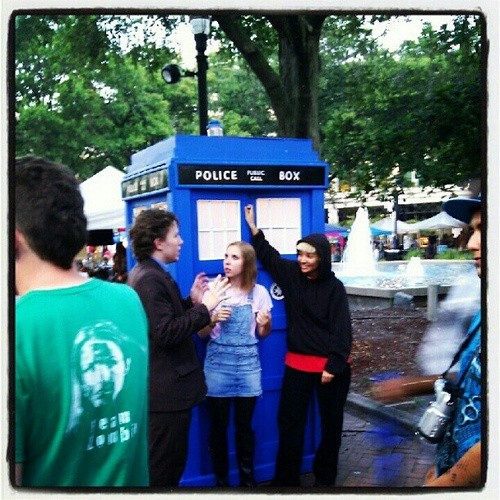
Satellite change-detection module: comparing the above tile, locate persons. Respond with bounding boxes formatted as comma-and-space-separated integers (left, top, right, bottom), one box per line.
419, 196, 480, 486
127, 209, 232, 485
198, 242, 274, 485
13, 154, 150, 485
245, 204, 353, 486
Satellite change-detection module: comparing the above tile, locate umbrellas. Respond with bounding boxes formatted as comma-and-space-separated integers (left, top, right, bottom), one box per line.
413, 211, 466, 237
370, 217, 415, 235
324, 223, 347, 232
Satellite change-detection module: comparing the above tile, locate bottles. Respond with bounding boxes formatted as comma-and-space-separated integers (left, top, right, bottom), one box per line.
206, 120, 223, 136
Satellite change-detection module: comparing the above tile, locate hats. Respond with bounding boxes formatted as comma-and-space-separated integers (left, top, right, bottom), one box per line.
296, 243, 316, 253
443, 191, 482, 223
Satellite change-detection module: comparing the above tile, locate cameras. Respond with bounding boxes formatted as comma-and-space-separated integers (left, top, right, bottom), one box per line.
416, 375, 465, 443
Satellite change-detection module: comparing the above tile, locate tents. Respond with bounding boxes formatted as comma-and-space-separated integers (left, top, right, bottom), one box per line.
79, 164, 128, 229
